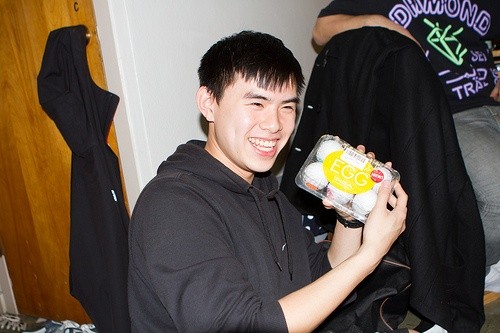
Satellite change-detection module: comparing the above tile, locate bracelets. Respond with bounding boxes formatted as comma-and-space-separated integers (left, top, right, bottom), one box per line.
335, 215, 364, 231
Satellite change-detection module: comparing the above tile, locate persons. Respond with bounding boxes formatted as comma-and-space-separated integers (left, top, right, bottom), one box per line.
311, 0, 500, 276
127, 29, 408, 333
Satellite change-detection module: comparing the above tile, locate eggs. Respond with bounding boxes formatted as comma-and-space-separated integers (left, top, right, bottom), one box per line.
303, 140, 392, 215
